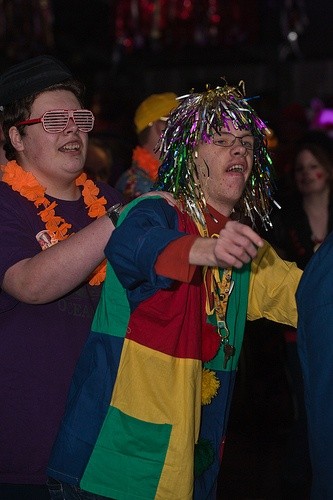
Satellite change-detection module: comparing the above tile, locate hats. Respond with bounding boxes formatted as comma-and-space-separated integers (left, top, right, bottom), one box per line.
133, 92, 181, 134
0, 55, 74, 105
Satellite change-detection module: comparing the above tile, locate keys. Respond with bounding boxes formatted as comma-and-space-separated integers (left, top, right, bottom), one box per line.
222, 336, 236, 370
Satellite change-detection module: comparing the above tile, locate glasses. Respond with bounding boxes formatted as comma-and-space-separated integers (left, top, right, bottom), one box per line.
206, 131, 261, 151
14, 109, 94, 134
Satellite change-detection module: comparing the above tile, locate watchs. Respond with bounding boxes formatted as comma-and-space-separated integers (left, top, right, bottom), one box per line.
106, 201, 124, 227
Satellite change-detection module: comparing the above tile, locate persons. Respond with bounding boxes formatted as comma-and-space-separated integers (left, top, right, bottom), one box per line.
46, 85, 304, 500
0, 71, 130, 500
83, 91, 193, 199
292, 140, 333, 266
294, 230, 333, 500
